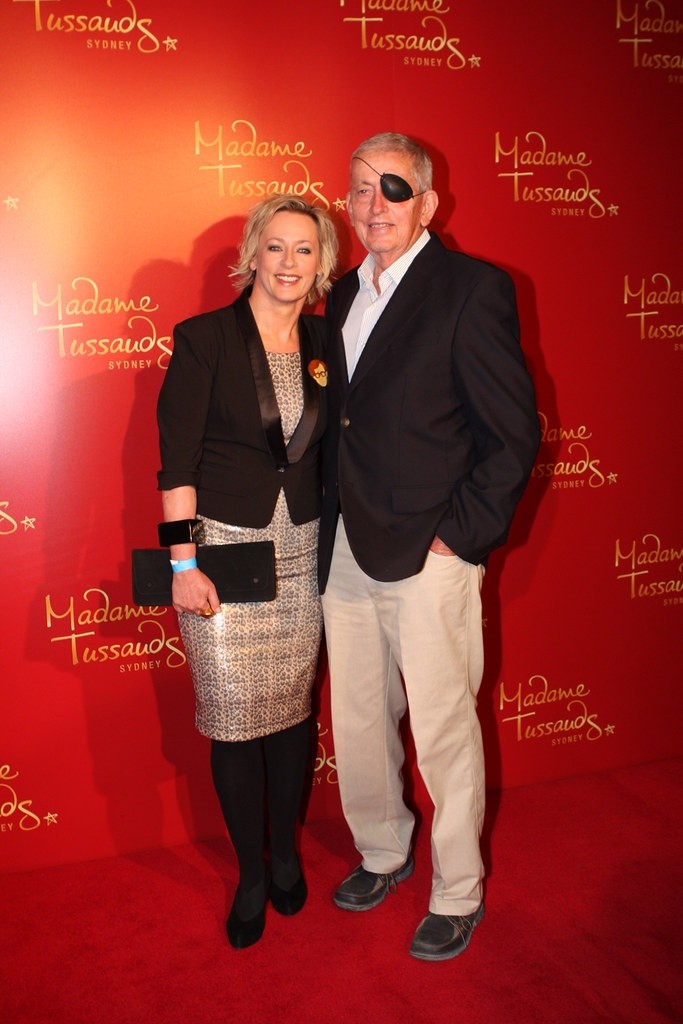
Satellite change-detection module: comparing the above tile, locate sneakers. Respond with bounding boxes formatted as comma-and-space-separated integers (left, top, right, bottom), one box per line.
408, 898, 485, 962
331, 853, 414, 912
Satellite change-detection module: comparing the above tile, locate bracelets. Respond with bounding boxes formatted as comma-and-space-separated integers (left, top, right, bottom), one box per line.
168, 558, 199, 572
160, 518, 207, 547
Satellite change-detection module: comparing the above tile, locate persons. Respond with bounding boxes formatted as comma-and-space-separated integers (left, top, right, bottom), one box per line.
319, 132, 539, 961
156, 193, 343, 951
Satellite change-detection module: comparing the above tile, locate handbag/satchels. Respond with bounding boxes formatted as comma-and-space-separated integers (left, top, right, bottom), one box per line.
131, 539, 277, 607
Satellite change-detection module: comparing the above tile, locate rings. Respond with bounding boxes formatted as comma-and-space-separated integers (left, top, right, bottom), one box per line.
201, 608, 214, 618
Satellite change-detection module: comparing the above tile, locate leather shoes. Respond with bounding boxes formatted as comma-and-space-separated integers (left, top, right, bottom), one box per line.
226, 853, 306, 949
